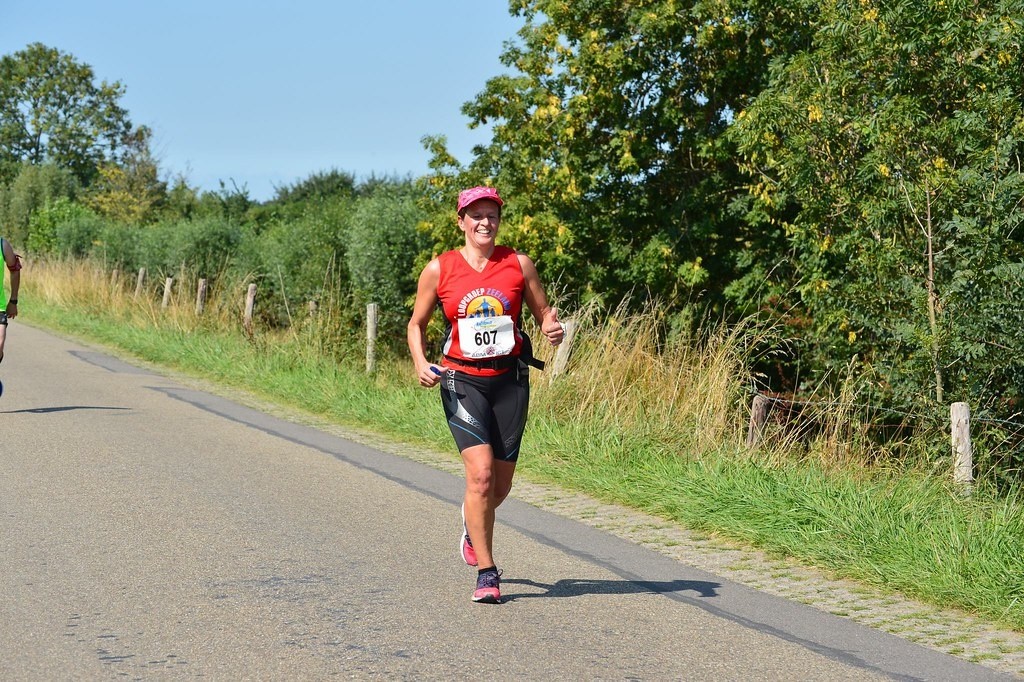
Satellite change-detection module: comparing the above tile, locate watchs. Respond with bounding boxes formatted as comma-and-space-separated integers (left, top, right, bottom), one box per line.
559, 321, 567, 338
9, 299, 18, 304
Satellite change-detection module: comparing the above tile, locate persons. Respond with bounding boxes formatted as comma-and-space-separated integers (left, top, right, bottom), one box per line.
0, 236, 22, 397
407, 187, 567, 604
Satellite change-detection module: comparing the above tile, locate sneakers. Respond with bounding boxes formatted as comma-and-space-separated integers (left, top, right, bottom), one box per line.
460, 503, 478, 566
471, 569, 503, 603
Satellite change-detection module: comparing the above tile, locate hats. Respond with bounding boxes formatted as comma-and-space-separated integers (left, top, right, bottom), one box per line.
457, 186, 503, 218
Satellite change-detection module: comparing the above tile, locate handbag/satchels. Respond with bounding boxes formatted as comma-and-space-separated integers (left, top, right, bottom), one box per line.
520, 330, 532, 365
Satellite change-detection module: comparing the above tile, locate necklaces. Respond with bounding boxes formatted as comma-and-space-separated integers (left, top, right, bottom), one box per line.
460, 250, 493, 270
